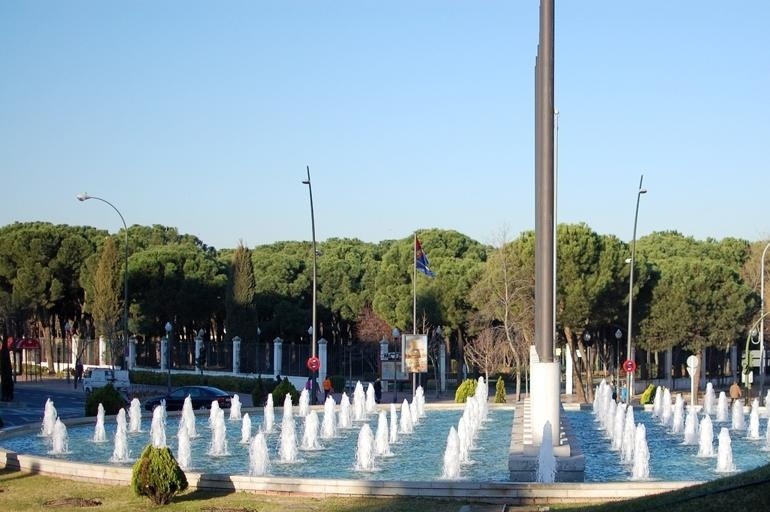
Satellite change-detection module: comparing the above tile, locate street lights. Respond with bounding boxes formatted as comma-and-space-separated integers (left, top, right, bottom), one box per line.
165, 322, 173, 391
626, 174, 648, 404
75, 191, 128, 371
582, 328, 592, 402
303, 165, 317, 405
63, 321, 72, 385
392, 329, 400, 403
615, 329, 622, 399
435, 325, 444, 393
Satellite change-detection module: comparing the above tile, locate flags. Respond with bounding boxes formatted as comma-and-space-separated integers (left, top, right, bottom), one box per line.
413, 237, 439, 279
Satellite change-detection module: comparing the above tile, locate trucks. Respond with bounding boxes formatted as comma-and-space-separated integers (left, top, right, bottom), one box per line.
82, 367, 130, 395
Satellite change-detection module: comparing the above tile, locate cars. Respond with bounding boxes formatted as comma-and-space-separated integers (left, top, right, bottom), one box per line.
145, 385, 234, 411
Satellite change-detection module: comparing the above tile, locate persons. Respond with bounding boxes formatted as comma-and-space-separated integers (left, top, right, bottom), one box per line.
315, 382, 322, 402
77, 359, 84, 383
611, 379, 617, 401
729, 380, 742, 408
622, 383, 628, 403
373, 375, 383, 402
323, 376, 332, 402
305, 374, 312, 404
